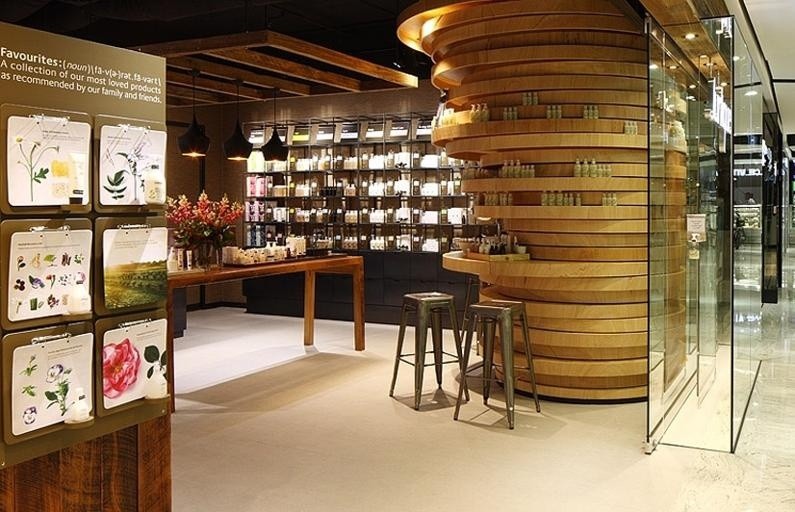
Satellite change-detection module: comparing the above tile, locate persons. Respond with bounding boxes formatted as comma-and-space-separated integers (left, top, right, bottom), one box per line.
744, 192, 756, 205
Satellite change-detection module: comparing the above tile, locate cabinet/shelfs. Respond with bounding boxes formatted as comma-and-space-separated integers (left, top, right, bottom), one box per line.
242, 111, 503, 330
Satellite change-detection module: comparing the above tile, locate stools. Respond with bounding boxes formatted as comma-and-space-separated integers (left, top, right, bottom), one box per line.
389, 290, 542, 430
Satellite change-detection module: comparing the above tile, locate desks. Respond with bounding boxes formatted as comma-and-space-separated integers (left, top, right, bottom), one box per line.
165, 254, 366, 413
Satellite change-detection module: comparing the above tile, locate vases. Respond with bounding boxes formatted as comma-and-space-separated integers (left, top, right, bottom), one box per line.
187, 233, 224, 274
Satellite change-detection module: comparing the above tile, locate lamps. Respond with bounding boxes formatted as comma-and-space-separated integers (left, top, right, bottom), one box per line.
176, 67, 290, 162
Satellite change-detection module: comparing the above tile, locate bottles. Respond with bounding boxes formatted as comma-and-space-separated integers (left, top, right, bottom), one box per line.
546, 104, 563, 119
521, 91, 539, 107
520, 164, 534, 177
540, 191, 582, 206
442, 108, 457, 126
69, 280, 92, 313
147, 360, 168, 399
502, 107, 518, 120
602, 192, 618, 206
369, 236, 385, 251
144, 163, 167, 206
582, 104, 599, 120
343, 236, 357, 249
623, 121, 638, 134
344, 183, 356, 196
574, 156, 612, 178
72, 387, 90, 423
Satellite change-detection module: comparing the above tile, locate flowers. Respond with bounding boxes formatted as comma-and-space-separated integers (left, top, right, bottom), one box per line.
166, 188, 247, 260
